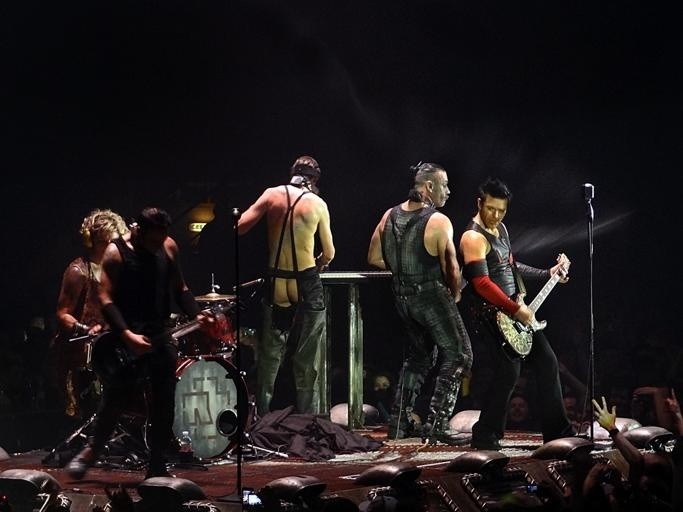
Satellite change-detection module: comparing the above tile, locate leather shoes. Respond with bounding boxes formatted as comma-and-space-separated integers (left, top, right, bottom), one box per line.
471, 440, 501, 450
423, 428, 474, 447
65, 445, 97, 480
143, 467, 177, 480
387, 423, 433, 439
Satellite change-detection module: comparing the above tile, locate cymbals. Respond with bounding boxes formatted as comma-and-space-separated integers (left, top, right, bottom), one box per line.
194, 293, 237, 302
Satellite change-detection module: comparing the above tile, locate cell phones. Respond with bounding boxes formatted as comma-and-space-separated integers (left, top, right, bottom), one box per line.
527, 485, 538, 493
242, 487, 254, 505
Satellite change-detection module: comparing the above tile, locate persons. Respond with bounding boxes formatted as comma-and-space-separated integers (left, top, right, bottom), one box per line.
236, 156, 336, 420
367, 162, 472, 445
67, 207, 202, 479
55, 208, 129, 422
460, 176, 578, 448
0, 315, 683, 512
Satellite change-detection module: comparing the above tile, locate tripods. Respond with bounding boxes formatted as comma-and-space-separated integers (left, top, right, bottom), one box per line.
42, 365, 153, 464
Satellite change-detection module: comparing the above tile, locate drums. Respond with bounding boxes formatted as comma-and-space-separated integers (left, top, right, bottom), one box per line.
119, 350, 255, 464
196, 305, 242, 359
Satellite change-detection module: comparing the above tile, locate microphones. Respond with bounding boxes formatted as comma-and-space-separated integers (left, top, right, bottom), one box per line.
582, 183, 594, 203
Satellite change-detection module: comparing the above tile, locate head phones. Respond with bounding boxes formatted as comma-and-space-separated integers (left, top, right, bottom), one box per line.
80, 208, 101, 248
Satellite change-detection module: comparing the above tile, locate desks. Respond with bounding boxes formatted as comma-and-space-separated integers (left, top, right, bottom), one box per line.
318, 270, 392, 426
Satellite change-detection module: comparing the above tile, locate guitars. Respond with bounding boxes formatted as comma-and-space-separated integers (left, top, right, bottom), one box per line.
491, 252, 572, 362
91, 302, 236, 392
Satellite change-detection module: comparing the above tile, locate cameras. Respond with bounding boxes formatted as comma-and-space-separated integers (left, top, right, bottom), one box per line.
248, 494, 262, 505
